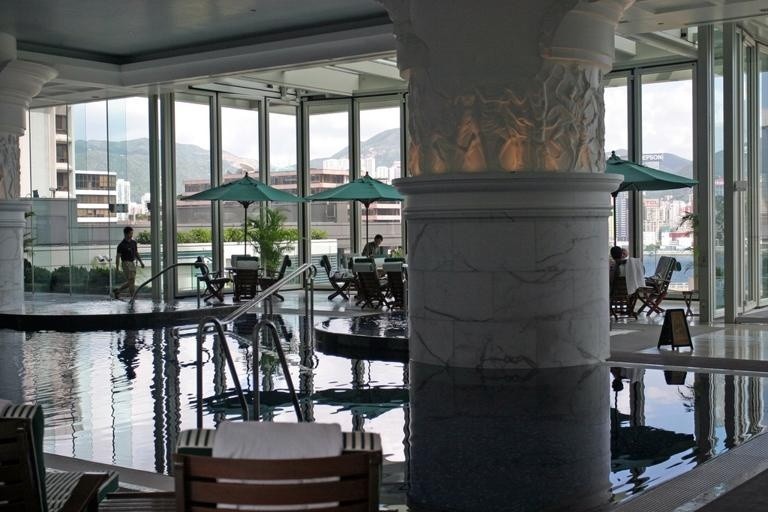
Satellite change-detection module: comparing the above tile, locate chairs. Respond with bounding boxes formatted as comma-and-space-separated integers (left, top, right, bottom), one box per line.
195, 255, 292, 302
318, 255, 410, 311
611, 256, 682, 321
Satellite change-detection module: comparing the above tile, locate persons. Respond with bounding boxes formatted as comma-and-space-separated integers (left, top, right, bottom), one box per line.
619, 248, 630, 261
609, 244, 627, 283
361, 234, 383, 259
115, 328, 146, 381
608, 366, 624, 391
111, 225, 146, 311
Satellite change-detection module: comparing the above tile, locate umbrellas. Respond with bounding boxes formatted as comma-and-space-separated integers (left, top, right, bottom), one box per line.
302, 171, 407, 259
609, 391, 698, 474
604, 150, 700, 248
309, 355, 409, 421
190, 358, 314, 420
180, 171, 312, 259
